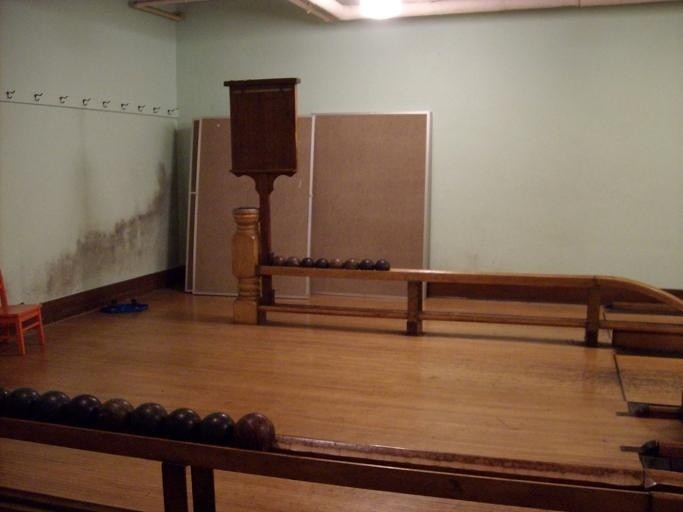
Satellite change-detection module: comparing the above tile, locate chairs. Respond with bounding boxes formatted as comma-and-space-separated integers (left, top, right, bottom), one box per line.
0, 268, 45, 355
